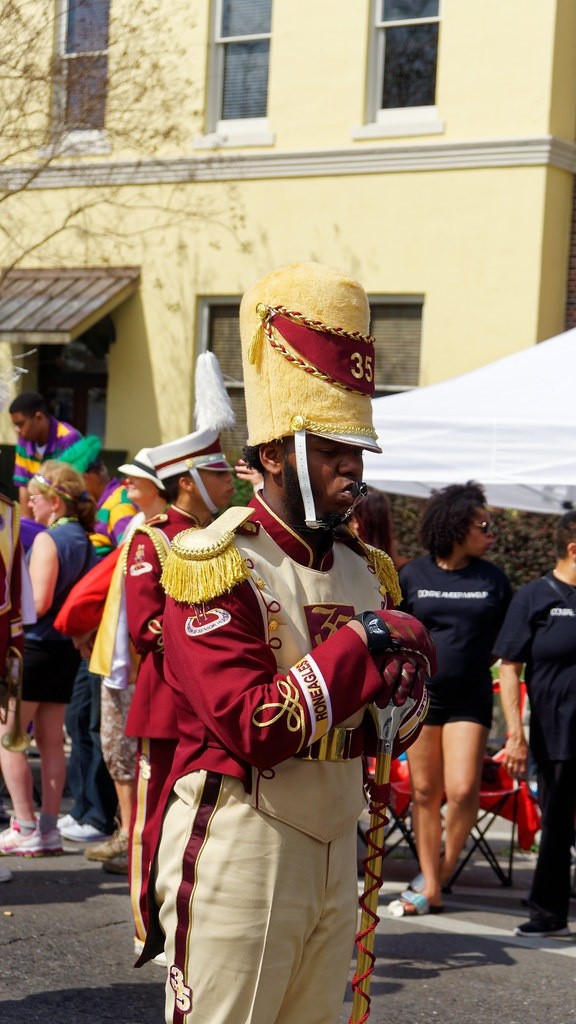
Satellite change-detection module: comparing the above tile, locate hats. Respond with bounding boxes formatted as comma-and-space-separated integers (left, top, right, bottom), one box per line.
119, 447, 166, 490
239, 265, 383, 457
147, 351, 234, 481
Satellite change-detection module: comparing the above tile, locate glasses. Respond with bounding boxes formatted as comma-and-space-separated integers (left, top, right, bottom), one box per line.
470, 522, 494, 533
30, 494, 45, 504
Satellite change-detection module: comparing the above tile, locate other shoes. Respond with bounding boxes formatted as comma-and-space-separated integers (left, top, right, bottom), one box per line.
0, 860, 10, 882
0, 799, 11, 823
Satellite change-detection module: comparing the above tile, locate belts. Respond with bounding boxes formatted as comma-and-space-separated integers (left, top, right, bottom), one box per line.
294, 726, 368, 762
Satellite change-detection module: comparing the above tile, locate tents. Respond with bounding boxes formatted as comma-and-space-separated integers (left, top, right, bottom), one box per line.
359, 321, 576, 514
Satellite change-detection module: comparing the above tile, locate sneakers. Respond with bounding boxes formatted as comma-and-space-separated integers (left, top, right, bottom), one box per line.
0, 816, 40, 856
60, 822, 114, 842
15, 821, 64, 857
55, 812, 79, 830
84, 817, 129, 861
102, 850, 128, 874
517, 918, 571, 937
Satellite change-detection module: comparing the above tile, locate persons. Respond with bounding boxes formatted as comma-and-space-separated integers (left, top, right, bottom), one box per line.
389, 482, 515, 918
88, 278, 438, 1024
0, 390, 166, 874
492, 511, 576, 937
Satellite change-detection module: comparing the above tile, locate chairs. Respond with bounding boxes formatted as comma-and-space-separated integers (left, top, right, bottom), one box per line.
354, 678, 543, 897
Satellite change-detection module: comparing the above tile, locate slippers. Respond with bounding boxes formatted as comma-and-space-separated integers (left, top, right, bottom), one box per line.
401, 875, 444, 915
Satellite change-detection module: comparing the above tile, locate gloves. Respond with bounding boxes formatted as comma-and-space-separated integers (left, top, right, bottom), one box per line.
5, 657, 20, 685
365, 648, 427, 709
353, 607, 438, 678
